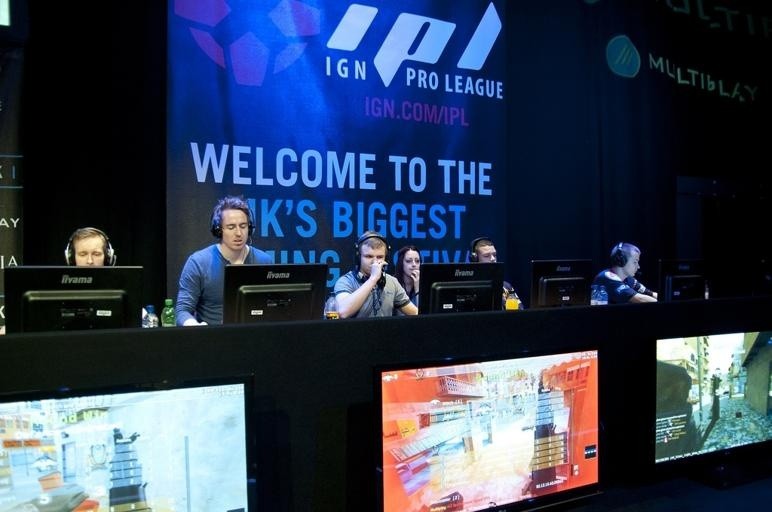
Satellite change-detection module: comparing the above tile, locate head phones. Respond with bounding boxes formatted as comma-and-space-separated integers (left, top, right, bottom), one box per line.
615, 242, 627, 265
353, 235, 393, 264
468, 238, 499, 261
209, 205, 256, 239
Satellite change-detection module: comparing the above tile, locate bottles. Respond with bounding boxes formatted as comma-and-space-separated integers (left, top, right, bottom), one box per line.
590, 285, 608, 305
324, 291, 340, 320
505, 288, 518, 309
142, 306, 158, 326
161, 300, 176, 328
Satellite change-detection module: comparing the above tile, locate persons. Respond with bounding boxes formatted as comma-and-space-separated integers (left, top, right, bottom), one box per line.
393, 246, 422, 316
175, 198, 272, 326
333, 232, 418, 319
591, 242, 658, 304
469, 236, 524, 311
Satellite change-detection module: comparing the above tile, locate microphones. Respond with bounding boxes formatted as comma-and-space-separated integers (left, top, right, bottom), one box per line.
637, 269, 643, 276
246, 236, 254, 245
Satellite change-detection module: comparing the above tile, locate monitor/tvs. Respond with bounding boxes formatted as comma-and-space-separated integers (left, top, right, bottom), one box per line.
415, 264, 505, 315
530, 260, 592, 308
3, 265, 143, 334
654, 328, 772, 492
2, 374, 256, 512
372, 346, 603, 512
223, 264, 329, 323
658, 260, 711, 303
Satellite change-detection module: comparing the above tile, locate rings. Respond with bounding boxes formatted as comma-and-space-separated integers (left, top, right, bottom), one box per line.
378, 261, 382, 264
